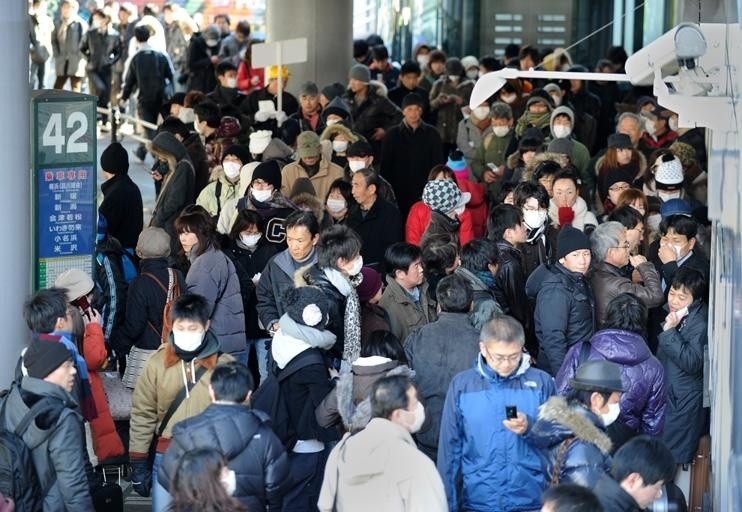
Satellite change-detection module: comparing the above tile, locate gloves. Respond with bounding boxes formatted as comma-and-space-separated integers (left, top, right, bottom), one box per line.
128, 460, 152, 497
255, 111, 288, 122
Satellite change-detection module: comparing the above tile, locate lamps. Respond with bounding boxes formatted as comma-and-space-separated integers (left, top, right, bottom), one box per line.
469, 67, 677, 111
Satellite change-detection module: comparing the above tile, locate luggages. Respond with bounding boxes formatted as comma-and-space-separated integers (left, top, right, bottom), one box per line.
646, 435, 711, 512
87, 465, 124, 512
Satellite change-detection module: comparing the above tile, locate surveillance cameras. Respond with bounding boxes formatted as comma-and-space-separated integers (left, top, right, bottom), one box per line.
624, 22, 708, 86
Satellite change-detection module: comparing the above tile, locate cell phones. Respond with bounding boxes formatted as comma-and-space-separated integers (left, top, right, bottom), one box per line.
78, 296, 95, 319
269, 330, 276, 335
142, 166, 158, 175
663, 305, 689, 332
506, 406, 517, 420
487, 162, 498, 172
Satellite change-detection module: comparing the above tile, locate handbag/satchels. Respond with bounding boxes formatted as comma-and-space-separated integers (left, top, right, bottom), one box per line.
121, 346, 158, 389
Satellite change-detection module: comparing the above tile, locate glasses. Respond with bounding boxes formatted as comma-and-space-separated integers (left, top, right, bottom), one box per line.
610, 243, 630, 248
523, 205, 546, 212
482, 346, 523, 363
608, 184, 630, 191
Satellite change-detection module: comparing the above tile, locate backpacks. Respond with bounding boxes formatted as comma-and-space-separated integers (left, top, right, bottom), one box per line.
96, 237, 138, 285
139, 269, 181, 344
251, 337, 326, 453
0, 393, 66, 512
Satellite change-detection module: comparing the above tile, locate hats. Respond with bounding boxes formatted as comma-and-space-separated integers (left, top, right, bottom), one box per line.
268, 64, 370, 100
659, 199, 693, 218
54, 268, 94, 302
278, 267, 382, 331
101, 143, 129, 175
401, 42, 480, 111
297, 131, 373, 159
569, 360, 626, 393
24, 333, 73, 378
556, 224, 591, 261
606, 133, 685, 191
637, 96, 656, 112
421, 150, 472, 214
135, 226, 172, 259
221, 145, 281, 189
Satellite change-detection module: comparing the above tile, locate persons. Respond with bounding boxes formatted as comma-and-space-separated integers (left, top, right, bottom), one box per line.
2, 1, 708, 511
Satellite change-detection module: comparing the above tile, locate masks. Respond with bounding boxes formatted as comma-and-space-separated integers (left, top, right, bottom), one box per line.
223, 162, 277, 202
340, 255, 364, 276
658, 242, 691, 263
238, 232, 263, 246
171, 328, 207, 351
594, 394, 621, 427
658, 191, 679, 202
206, 39, 237, 89
454, 204, 465, 216
399, 400, 426, 433
473, 93, 517, 138
218, 470, 236, 497
552, 93, 572, 139
522, 205, 547, 228
417, 54, 479, 82
326, 141, 368, 212
